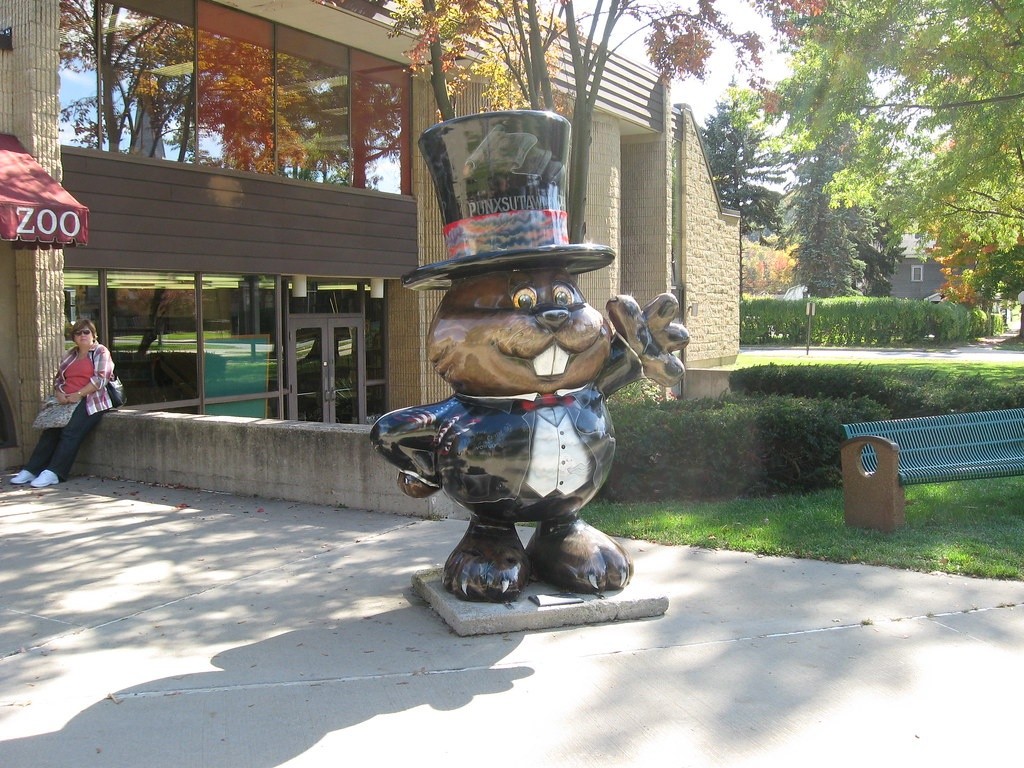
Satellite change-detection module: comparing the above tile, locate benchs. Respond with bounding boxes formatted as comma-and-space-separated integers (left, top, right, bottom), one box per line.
839, 408, 1024, 533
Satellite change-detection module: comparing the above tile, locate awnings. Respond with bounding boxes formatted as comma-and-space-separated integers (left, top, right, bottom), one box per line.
0, 134, 90, 251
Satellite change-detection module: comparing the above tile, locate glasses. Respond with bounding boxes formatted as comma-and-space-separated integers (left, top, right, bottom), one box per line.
73, 330, 91, 336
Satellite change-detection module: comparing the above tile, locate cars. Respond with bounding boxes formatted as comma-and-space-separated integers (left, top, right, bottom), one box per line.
922, 292, 944, 305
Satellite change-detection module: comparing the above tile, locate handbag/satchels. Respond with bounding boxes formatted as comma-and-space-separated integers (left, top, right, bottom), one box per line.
32, 394, 80, 429
105, 370, 128, 409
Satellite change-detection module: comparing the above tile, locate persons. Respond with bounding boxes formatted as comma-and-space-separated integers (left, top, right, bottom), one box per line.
9, 318, 116, 488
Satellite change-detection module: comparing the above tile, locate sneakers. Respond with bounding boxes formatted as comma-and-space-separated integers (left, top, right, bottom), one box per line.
9, 469, 37, 484
30, 470, 59, 488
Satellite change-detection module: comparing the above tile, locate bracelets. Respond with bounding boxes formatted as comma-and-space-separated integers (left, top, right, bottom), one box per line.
77, 391, 82, 396
